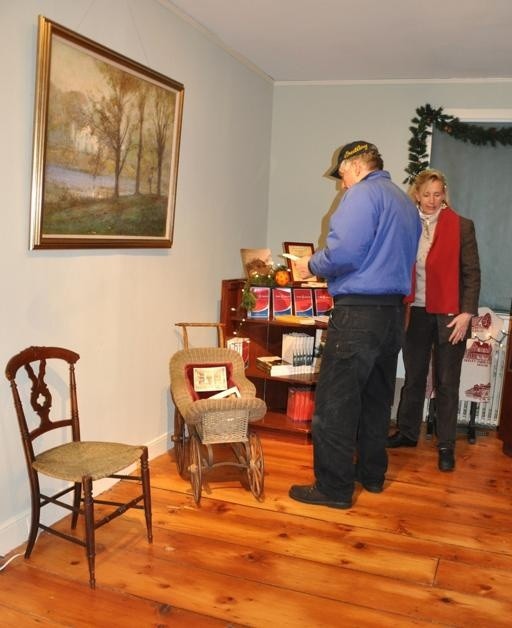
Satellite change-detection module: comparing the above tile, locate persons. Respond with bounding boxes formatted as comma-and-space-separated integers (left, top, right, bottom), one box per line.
289, 141, 422, 510
384, 166, 483, 472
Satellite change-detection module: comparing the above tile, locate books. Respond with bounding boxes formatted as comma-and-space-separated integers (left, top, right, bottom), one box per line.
300, 281, 327, 287
191, 365, 228, 393
206, 385, 241, 399
280, 251, 301, 261
226, 285, 338, 423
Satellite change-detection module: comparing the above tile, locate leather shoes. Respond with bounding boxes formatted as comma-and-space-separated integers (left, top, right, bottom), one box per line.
385, 429, 418, 449
353, 462, 385, 493
437, 447, 456, 472
288, 482, 353, 509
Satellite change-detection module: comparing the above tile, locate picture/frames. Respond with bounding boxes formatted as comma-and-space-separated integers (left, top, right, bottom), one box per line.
281, 239, 321, 287
26, 13, 185, 251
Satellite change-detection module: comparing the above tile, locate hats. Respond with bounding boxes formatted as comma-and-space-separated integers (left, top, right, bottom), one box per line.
329, 140, 378, 179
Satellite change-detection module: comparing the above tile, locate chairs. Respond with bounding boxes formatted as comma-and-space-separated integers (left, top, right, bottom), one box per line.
3, 345, 155, 591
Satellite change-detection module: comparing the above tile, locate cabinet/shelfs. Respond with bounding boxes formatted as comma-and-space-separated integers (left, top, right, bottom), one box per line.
216, 277, 328, 443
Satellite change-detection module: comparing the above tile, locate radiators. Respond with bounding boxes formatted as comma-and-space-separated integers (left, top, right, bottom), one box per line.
426, 307, 511, 430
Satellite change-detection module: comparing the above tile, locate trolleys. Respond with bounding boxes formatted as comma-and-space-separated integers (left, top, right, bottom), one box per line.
171, 320, 266, 503
